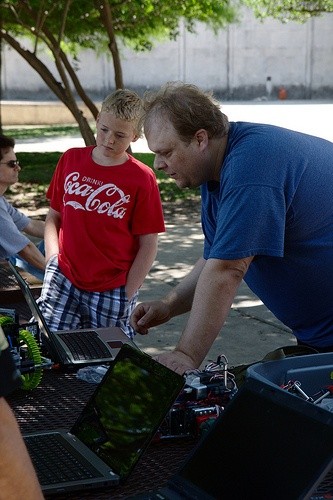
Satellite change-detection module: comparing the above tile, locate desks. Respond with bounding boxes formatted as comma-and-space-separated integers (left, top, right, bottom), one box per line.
0, 364, 333, 500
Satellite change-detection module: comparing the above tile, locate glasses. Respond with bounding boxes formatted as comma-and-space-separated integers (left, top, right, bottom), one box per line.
0, 160, 19, 168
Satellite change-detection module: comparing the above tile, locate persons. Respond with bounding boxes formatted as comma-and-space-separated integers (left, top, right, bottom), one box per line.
129, 81, 333, 376
266, 76, 274, 100
278, 85, 286, 100
0, 326, 45, 500
25, 88, 166, 368
0, 136, 46, 286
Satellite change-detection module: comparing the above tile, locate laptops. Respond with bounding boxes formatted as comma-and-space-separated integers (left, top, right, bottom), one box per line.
19, 341, 186, 494
6, 257, 139, 369
123, 376, 333, 500
